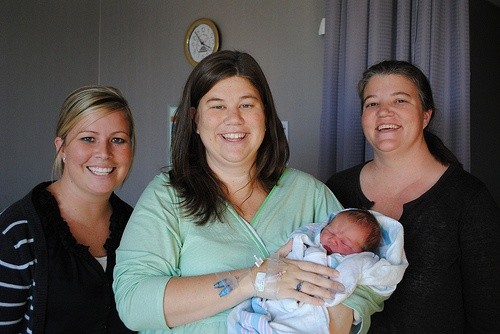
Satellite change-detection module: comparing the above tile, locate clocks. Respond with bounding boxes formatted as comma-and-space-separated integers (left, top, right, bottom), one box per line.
183, 17, 222, 67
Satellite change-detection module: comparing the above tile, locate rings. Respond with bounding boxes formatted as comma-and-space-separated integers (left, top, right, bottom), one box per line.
296, 281, 304, 291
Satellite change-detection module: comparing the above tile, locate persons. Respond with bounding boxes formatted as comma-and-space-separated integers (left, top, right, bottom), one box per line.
114, 51, 385, 334
0, 83, 137, 334
228, 209, 379, 333
324, 62, 500, 334
